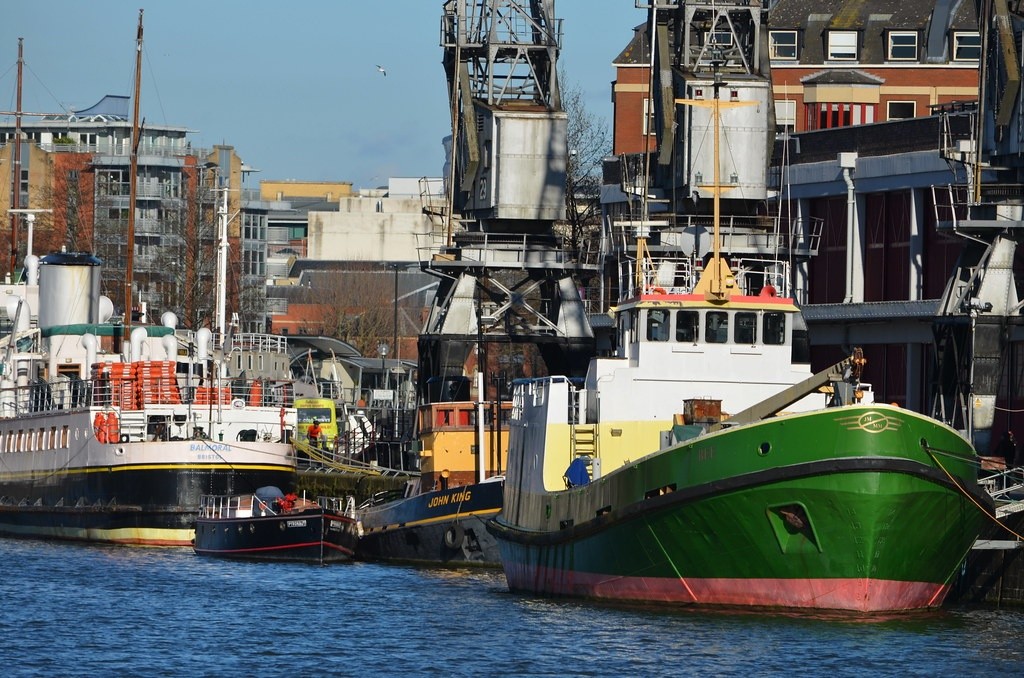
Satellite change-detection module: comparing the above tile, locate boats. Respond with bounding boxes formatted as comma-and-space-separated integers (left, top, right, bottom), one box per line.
248, 380, 263, 407
351, 372, 514, 567
192, 484, 360, 566
94, 411, 119, 445
466, 1, 994, 620
88, 360, 182, 410
193, 385, 230, 405
0, 9, 297, 548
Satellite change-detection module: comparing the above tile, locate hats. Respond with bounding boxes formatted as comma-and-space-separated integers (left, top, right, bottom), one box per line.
313, 421, 320, 425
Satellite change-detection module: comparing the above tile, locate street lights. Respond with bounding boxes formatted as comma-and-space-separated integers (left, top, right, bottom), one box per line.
378, 344, 390, 407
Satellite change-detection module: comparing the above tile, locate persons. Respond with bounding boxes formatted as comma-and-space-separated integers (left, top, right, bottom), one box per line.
307, 421, 322, 447
994, 432, 1015, 497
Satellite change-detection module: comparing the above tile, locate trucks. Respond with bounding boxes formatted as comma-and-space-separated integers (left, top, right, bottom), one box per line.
294, 396, 339, 462
